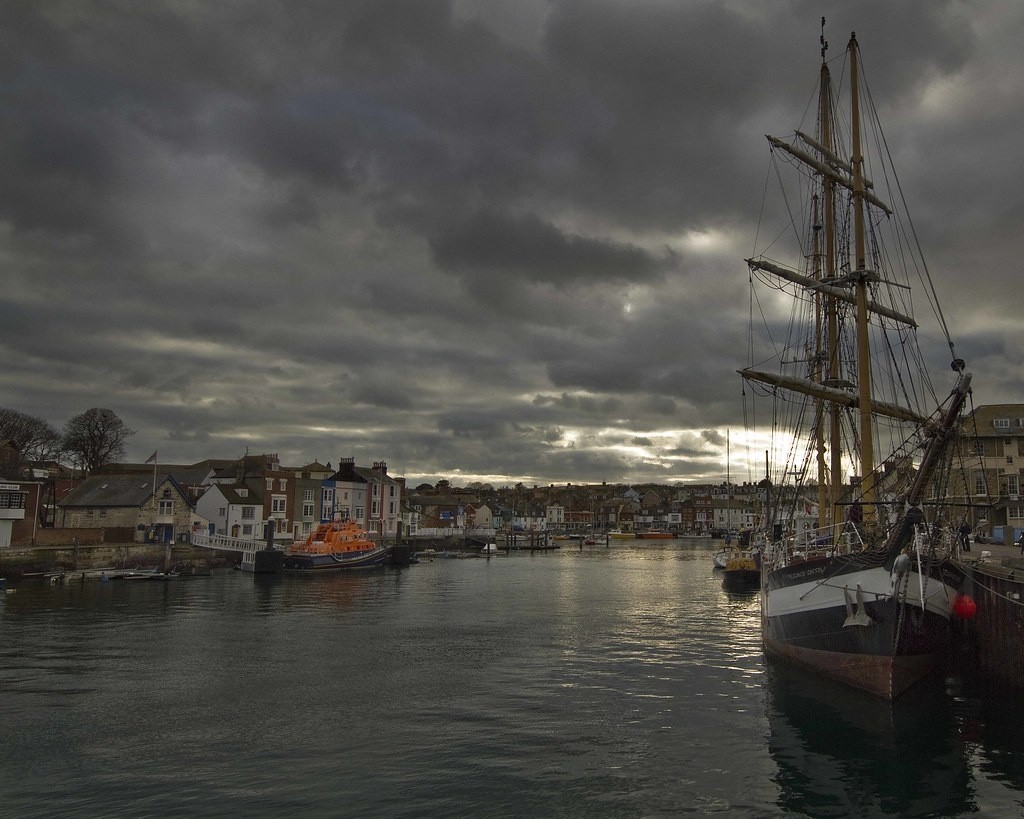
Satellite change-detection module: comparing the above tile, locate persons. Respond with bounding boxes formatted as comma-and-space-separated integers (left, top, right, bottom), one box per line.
101, 572, 105, 577
751, 545, 758, 554
960, 522, 970, 535
147, 529, 156, 542
382, 520, 387, 536
345, 508, 350, 523
1020, 532, 1024, 555
340, 507, 345, 523
377, 519, 382, 536
747, 543, 753, 551
550, 534, 552, 537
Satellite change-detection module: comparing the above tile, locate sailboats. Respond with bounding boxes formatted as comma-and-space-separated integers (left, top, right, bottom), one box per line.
711, 426, 774, 579
734, 14, 1002, 704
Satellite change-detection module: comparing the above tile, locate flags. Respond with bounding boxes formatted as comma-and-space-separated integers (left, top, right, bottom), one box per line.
806, 506, 811, 514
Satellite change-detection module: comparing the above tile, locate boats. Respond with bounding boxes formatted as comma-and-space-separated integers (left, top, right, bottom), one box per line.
496, 532, 607, 545
608, 529, 636, 539
483, 544, 496, 555
637, 529, 678, 539
285, 520, 393, 571
